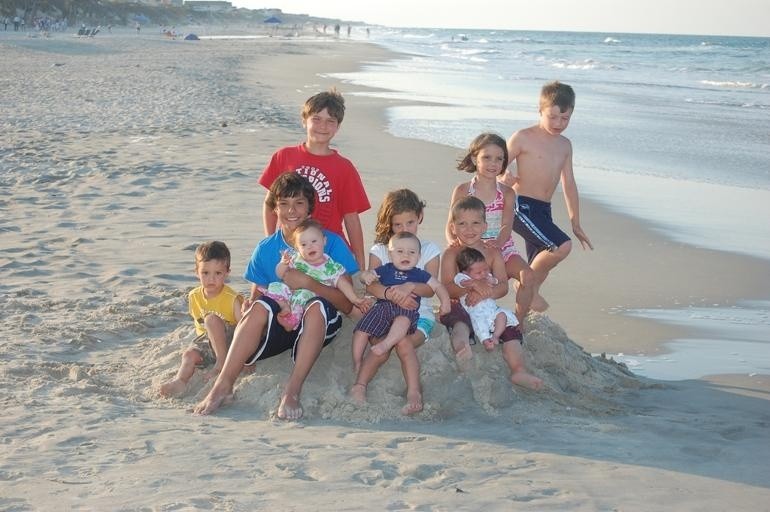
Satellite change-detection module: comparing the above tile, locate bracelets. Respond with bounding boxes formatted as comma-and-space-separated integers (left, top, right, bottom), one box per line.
384, 286, 391, 301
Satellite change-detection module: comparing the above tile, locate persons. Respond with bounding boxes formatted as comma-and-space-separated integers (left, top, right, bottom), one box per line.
348, 188, 443, 415
453, 245, 519, 353
352, 231, 452, 376
439, 195, 543, 391
0, 12, 143, 38
445, 132, 537, 334
258, 85, 370, 270
190, 169, 360, 420
264, 220, 372, 332
496, 80, 594, 316
159, 240, 246, 395
